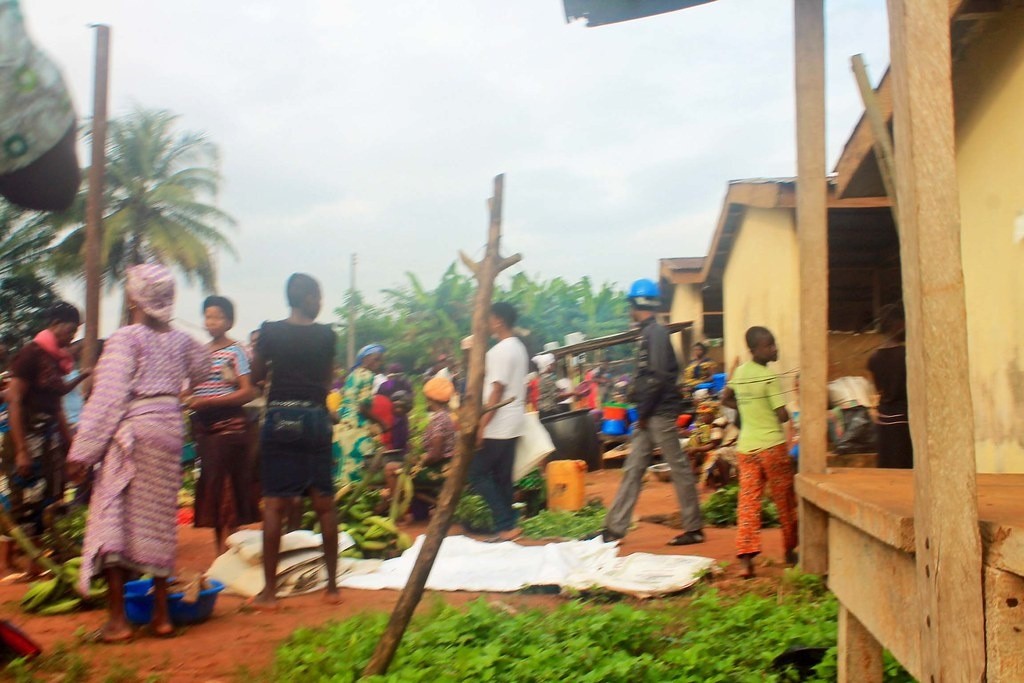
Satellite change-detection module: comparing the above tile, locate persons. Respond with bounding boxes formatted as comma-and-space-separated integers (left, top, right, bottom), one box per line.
579, 278, 707, 546
864, 301, 916, 468
374, 375, 460, 533
720, 325, 801, 578
471, 300, 532, 540
61, 260, 211, 646
331, 343, 390, 516
239, 272, 343, 613
242, 328, 802, 463
0, 301, 111, 572
177, 293, 257, 551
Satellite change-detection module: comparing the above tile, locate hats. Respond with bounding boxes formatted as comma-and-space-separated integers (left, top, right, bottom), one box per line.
423, 378, 456, 404
624, 280, 659, 297
126, 264, 174, 324
48, 300, 79, 320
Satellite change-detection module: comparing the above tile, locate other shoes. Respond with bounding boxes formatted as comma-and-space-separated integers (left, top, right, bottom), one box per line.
578, 530, 624, 546
733, 553, 798, 577
667, 531, 706, 544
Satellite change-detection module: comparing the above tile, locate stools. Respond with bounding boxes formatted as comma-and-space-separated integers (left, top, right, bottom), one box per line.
412, 488, 439, 521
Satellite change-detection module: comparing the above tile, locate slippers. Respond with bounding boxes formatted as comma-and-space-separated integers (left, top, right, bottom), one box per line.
87, 626, 177, 643
238, 593, 345, 611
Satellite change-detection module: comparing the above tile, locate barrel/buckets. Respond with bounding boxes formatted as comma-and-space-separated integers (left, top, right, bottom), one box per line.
539, 406, 601, 472
546, 459, 589, 513
